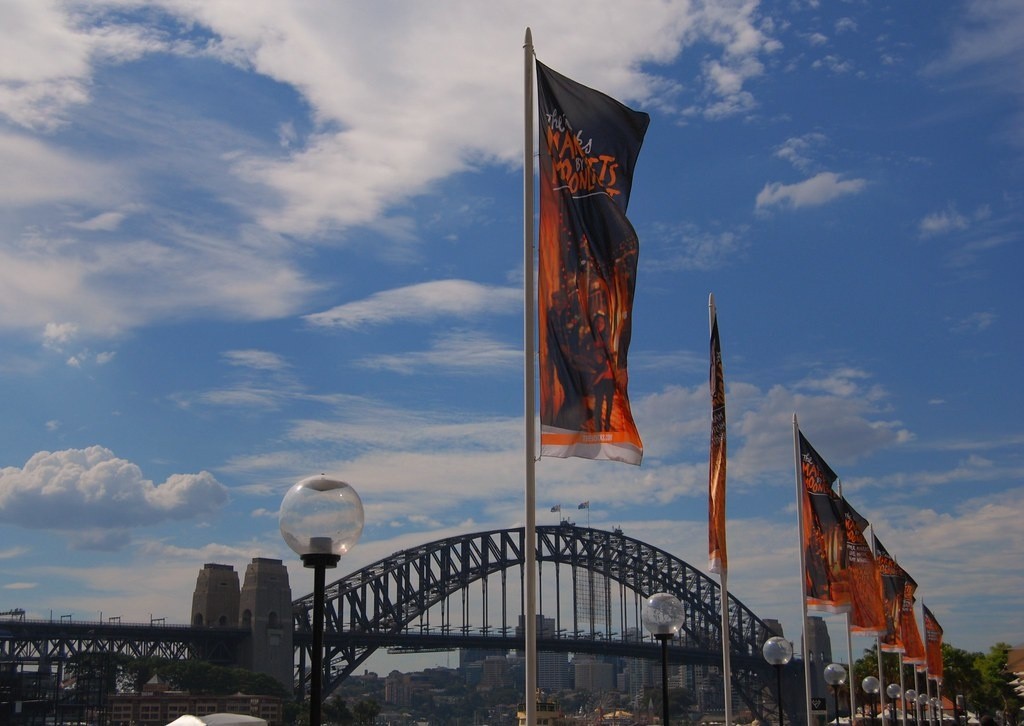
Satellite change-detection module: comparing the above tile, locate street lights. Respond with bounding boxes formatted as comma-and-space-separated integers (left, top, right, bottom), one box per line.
277, 475, 364, 726
919, 694, 929, 725
929, 697, 942, 725
905, 690, 918, 726
642, 594, 685, 726
763, 636, 794, 726
824, 664, 847, 726
862, 677, 879, 726
887, 684, 901, 726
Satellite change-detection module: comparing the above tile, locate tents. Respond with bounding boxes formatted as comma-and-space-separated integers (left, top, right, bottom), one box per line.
876, 693, 982, 726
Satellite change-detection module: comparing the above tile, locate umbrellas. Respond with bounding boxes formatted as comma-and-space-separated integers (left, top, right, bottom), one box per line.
830, 708, 955, 726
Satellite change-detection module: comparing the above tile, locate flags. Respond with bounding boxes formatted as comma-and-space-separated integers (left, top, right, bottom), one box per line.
872, 534, 906, 653
923, 605, 943, 682
840, 495, 887, 635
901, 572, 928, 672
708, 309, 727, 583
797, 429, 852, 617
535, 59, 650, 466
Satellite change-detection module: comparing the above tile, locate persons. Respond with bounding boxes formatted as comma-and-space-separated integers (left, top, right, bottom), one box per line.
883, 599, 898, 644
547, 291, 619, 433
806, 535, 834, 601
